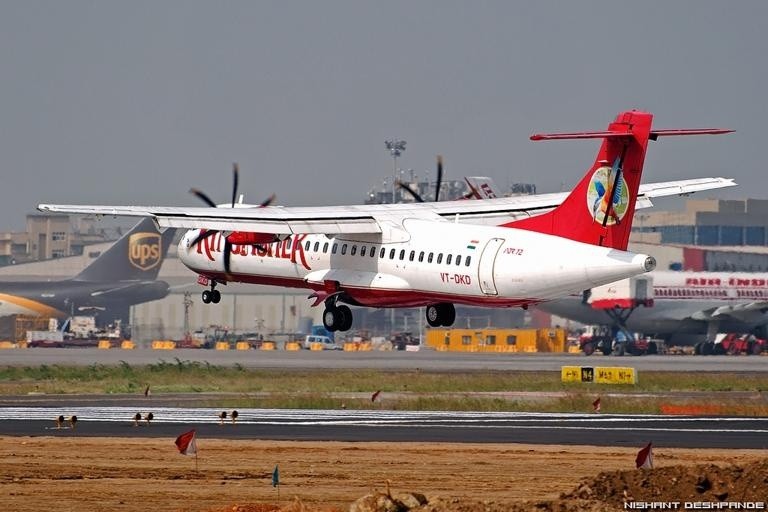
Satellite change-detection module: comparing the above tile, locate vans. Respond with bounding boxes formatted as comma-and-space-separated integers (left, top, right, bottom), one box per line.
304, 334, 344, 350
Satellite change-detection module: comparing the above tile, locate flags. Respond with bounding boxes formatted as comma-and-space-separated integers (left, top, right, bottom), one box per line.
143, 385, 155, 398
592, 397, 601, 411
270, 466, 280, 487
634, 441, 654, 469
372, 390, 381, 404
173, 429, 198, 457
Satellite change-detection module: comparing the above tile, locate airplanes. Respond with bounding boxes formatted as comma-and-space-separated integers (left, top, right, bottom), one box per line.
0, 216, 177, 324
533, 269, 768, 357
32, 106, 739, 335
